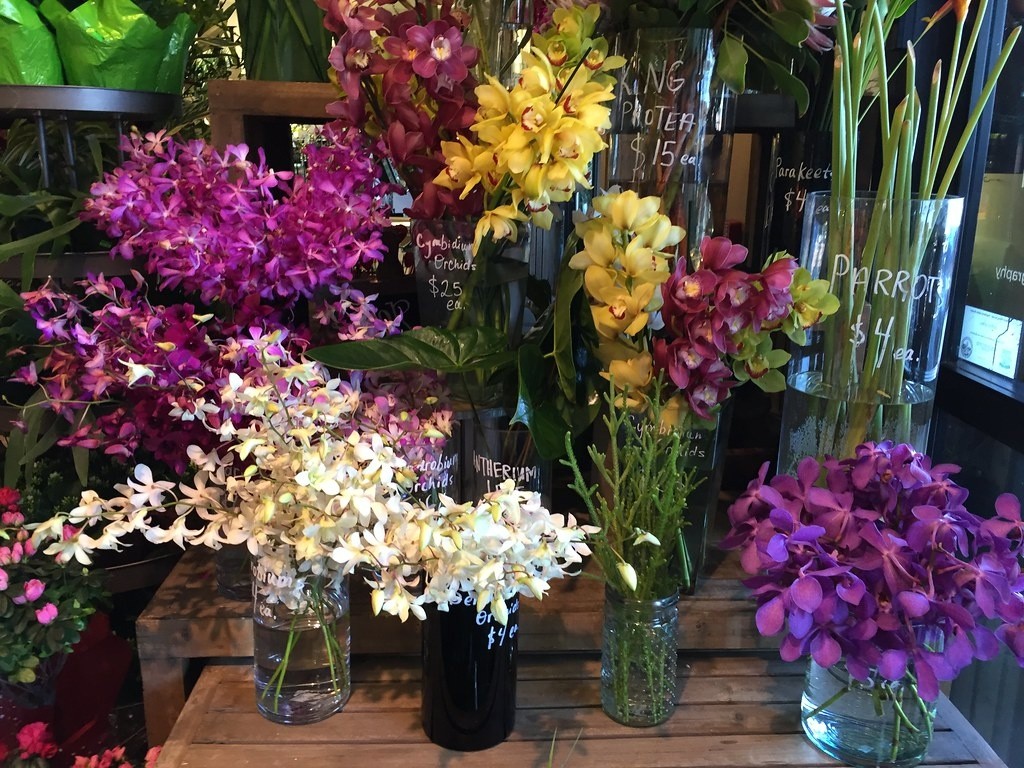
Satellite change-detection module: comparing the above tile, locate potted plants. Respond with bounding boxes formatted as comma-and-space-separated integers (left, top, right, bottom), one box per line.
559, 367, 709, 727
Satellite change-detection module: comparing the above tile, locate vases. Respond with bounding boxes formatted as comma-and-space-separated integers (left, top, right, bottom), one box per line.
777, 192, 965, 490
421, 569, 519, 752
800, 646, 937, 768
251, 551, 351, 726
569, 29, 717, 276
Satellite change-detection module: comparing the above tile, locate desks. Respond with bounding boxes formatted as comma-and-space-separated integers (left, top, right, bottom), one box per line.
136, 540, 793, 747
153, 654, 1007, 768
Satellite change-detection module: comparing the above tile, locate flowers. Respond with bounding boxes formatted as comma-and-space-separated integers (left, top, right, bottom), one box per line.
0, 0, 1024, 768
